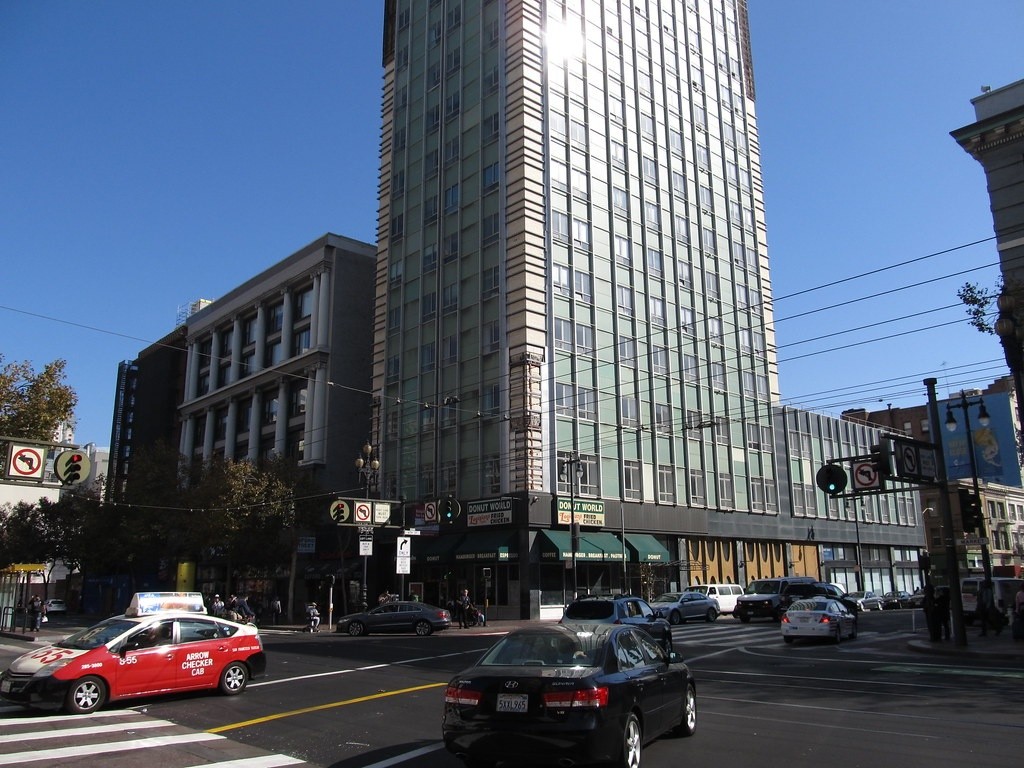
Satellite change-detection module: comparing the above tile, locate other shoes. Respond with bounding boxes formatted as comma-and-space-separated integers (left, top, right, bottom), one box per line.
459, 625, 462, 629
30, 628, 34, 631
36, 628, 40, 631
464, 626, 470, 629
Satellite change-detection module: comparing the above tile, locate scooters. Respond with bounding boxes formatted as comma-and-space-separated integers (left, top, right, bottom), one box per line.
303, 612, 321, 633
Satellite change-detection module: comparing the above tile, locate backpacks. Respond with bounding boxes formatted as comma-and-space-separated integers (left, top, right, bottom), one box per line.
225, 596, 242, 610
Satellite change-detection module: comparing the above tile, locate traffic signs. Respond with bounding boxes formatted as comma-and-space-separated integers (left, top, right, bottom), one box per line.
397, 536, 411, 556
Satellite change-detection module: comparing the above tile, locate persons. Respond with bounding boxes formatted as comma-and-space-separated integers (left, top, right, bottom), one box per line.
69, 585, 80, 615
921, 584, 938, 642
935, 587, 951, 641
456, 589, 470, 629
407, 592, 418, 603
915, 587, 920, 595
305, 602, 320, 630
378, 589, 390, 605
270, 596, 281, 626
470, 602, 491, 626
975, 579, 1002, 637
1014, 584, 1024, 618
28, 593, 45, 632
203, 592, 255, 620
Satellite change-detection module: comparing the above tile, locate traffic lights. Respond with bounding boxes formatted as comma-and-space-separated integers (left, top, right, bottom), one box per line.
329, 500, 349, 522
870, 438, 892, 474
438, 498, 461, 521
816, 464, 848, 495
958, 488, 981, 533
57, 449, 91, 484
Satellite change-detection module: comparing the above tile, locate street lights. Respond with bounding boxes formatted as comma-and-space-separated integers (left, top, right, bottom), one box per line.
355, 439, 380, 613
845, 496, 866, 592
559, 450, 583, 602
946, 388, 994, 607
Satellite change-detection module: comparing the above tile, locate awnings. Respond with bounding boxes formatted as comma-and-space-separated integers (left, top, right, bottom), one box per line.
393, 534, 462, 561
540, 529, 603, 561
620, 534, 670, 562
456, 530, 519, 562
579, 531, 630, 561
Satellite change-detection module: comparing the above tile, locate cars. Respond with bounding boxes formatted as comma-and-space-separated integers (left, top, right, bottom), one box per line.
848, 590, 885, 612
336, 601, 452, 637
0, 592, 267, 716
882, 591, 910, 609
780, 595, 857, 644
442, 623, 697, 768
646, 592, 721, 625
907, 588, 926, 609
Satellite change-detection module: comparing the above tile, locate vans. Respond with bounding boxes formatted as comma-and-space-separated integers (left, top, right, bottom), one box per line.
684, 583, 744, 616
959, 576, 1024, 627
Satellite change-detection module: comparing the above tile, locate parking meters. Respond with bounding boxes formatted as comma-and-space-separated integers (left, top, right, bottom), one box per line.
324, 574, 335, 630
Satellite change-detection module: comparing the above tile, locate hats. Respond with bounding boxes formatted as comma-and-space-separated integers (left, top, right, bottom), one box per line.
312, 602, 317, 606
215, 594, 219, 598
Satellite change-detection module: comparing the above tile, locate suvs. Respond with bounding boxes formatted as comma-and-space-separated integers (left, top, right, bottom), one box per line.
778, 582, 858, 623
558, 593, 673, 654
46, 598, 66, 617
737, 576, 815, 623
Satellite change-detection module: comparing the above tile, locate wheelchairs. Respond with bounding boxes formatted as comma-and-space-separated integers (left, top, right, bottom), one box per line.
467, 609, 478, 626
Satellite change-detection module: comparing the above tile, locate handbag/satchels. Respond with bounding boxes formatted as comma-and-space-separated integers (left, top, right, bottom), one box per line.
42, 616, 48, 623
